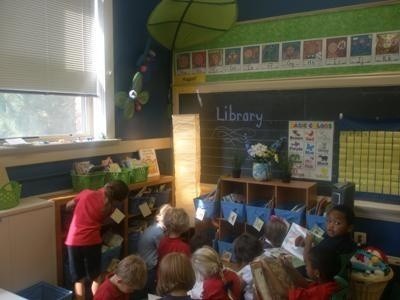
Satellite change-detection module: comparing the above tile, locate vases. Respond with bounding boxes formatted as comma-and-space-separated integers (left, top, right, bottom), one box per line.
252, 160, 273, 182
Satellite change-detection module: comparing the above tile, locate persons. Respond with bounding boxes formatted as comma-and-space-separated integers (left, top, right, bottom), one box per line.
64, 179, 130, 300
261, 213, 288, 249
302, 203, 356, 278
152, 206, 193, 294
154, 252, 197, 300
137, 203, 173, 289
191, 245, 243, 300
93, 254, 148, 300
230, 234, 266, 300
252, 243, 342, 300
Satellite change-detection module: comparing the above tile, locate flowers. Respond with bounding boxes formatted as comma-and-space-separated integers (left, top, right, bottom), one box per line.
243, 142, 280, 165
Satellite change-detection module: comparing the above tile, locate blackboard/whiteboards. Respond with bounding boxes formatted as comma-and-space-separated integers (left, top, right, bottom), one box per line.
178, 85, 400, 204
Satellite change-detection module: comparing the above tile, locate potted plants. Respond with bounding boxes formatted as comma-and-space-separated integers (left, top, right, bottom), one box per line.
230, 144, 244, 179
277, 153, 301, 183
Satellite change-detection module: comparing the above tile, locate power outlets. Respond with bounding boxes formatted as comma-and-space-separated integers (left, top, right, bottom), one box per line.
353, 231, 368, 246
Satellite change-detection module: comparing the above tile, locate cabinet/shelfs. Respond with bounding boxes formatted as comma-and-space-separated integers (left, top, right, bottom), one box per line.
217, 175, 318, 264
37, 174, 176, 296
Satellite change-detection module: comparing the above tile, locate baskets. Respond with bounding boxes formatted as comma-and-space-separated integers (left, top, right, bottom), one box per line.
105, 169, 131, 186
72, 172, 106, 192
352, 267, 394, 300
0, 181, 22, 210
121, 161, 148, 183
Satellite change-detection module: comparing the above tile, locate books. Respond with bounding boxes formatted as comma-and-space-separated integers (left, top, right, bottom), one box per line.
222, 192, 244, 204
263, 222, 325, 272
138, 148, 160, 178
314, 197, 333, 218
264, 194, 275, 208
291, 203, 305, 213
249, 253, 297, 299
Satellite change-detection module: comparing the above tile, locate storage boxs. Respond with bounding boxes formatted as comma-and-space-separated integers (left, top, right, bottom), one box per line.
110, 242, 123, 258
273, 201, 306, 225
244, 199, 274, 225
64, 246, 113, 290
305, 206, 330, 231
129, 197, 151, 214
15, 279, 72, 299
217, 233, 240, 262
220, 199, 247, 224
193, 193, 220, 220
112, 221, 139, 254
143, 188, 172, 206
